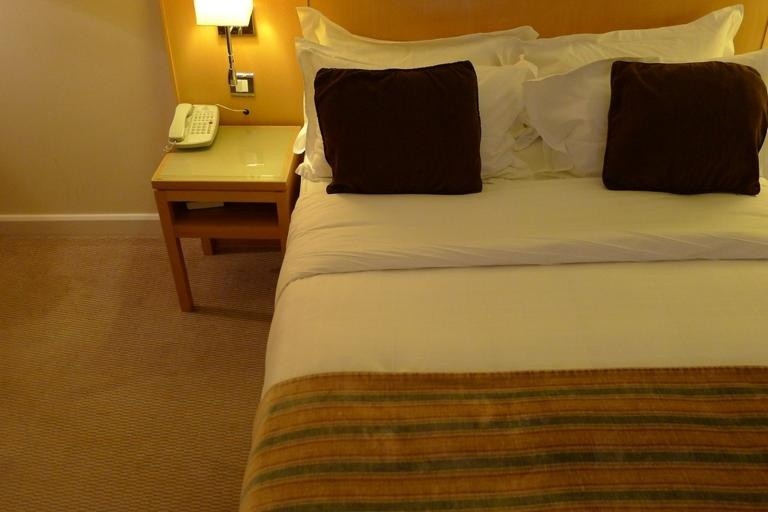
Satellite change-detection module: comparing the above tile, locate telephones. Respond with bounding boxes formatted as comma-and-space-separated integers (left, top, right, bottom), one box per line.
168, 103, 220, 148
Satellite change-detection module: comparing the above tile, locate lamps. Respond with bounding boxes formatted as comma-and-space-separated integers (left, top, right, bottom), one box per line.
193, 1, 257, 87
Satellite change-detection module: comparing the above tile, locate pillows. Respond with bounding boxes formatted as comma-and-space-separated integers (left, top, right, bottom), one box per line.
292, 34, 538, 184
312, 58, 485, 195
295, 5, 541, 154
479, 5, 746, 75
601, 57, 767, 196
521, 47, 768, 175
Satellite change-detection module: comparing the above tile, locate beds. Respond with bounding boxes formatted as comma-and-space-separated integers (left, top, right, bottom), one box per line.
238, 174, 768, 512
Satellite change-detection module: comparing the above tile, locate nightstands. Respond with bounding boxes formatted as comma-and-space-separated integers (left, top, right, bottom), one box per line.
150, 125, 304, 312
